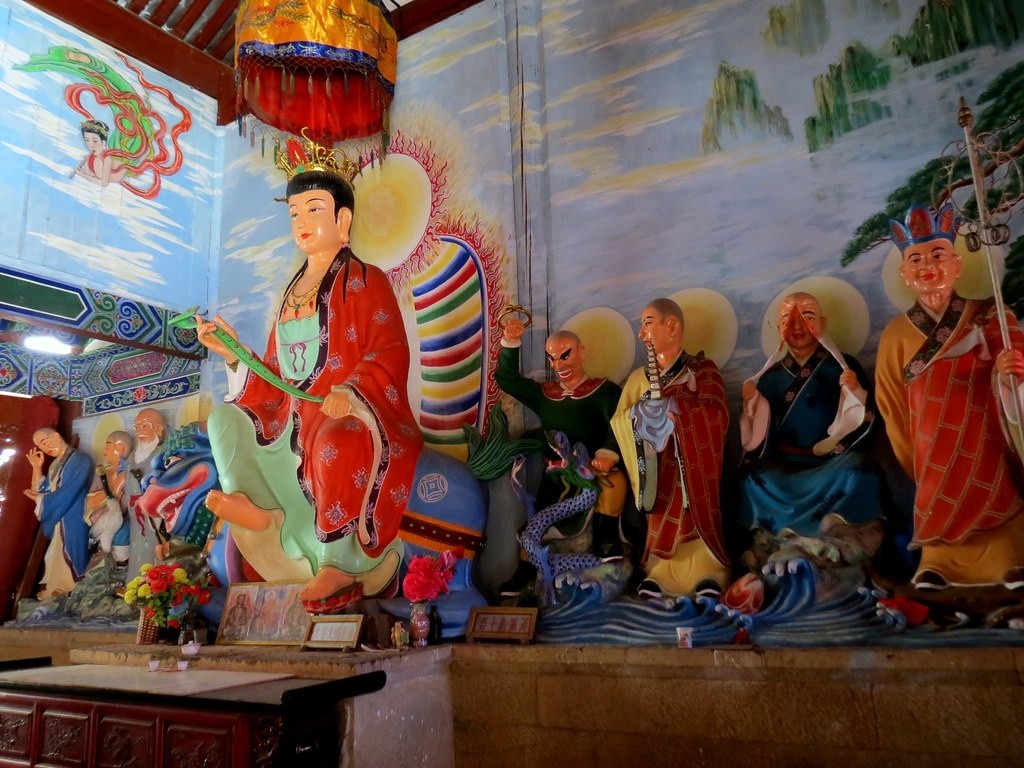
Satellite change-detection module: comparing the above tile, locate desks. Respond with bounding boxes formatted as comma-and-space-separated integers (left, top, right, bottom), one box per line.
1, 666, 388, 768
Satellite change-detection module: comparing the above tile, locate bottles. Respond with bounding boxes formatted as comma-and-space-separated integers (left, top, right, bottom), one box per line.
428, 605, 442, 646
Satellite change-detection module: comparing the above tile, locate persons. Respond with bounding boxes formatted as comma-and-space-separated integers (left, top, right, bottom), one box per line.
22, 168, 1024, 617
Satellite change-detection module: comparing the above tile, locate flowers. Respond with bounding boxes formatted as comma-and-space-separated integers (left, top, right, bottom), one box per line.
121, 561, 220, 627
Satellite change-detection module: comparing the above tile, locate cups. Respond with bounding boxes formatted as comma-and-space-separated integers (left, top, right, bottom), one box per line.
148, 661, 160, 669
193, 629, 207, 646
677, 625, 693, 648
177, 661, 188, 670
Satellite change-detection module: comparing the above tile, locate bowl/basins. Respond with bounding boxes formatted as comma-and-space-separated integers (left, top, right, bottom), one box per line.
182, 641, 200, 655
